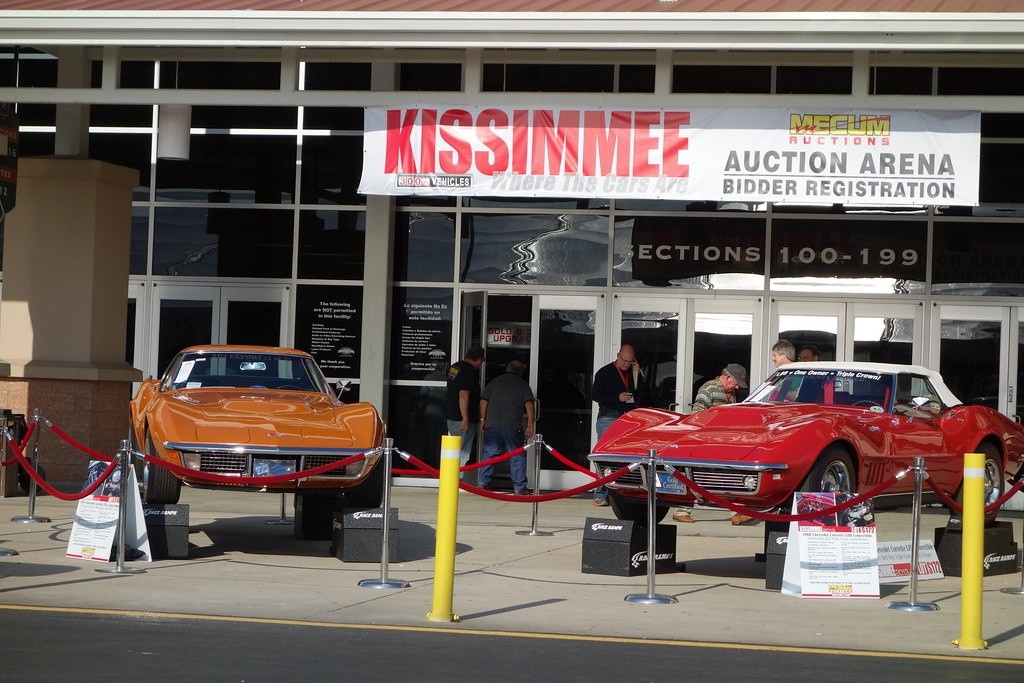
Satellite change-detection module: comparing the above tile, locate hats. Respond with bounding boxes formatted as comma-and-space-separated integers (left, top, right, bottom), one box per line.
726, 363, 748, 388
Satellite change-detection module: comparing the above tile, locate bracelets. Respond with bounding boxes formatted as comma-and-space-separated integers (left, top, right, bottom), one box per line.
480, 418, 486, 421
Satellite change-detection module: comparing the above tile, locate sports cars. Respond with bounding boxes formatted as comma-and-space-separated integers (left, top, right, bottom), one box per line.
126, 344, 389, 508
587, 360, 1023, 526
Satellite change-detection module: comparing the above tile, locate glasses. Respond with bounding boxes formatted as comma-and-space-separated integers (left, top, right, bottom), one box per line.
619, 354, 635, 364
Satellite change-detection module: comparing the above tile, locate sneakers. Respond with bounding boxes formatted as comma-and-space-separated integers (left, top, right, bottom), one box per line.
593, 496, 610, 506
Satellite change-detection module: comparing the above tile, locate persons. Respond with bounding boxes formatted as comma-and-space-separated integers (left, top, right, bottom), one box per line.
764, 339, 806, 402
591, 344, 649, 507
441, 346, 487, 482
476, 360, 536, 495
416, 358, 448, 469
671, 364, 756, 527
797, 345, 820, 362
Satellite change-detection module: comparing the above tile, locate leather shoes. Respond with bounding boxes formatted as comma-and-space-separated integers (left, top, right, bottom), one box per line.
478, 484, 498, 491
672, 514, 695, 523
732, 513, 756, 526
514, 487, 533, 496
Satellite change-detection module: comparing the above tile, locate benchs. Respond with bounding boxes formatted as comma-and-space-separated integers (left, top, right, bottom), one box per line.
814, 390, 916, 408
176, 374, 307, 392
